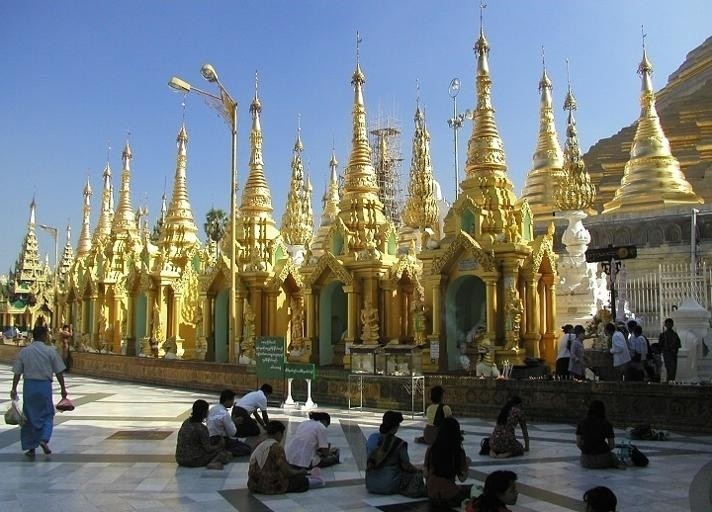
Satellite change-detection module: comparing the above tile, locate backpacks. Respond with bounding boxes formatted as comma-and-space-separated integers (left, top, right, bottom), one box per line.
618, 439, 635, 467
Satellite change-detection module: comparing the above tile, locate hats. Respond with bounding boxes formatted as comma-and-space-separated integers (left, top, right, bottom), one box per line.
441, 416, 464, 438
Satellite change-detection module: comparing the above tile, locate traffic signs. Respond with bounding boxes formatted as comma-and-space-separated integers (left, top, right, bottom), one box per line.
586, 246, 640, 263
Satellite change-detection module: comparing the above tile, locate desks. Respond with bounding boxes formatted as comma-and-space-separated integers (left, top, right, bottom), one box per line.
348, 373, 425, 420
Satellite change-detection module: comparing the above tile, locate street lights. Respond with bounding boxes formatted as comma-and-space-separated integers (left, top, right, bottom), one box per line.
167, 63, 240, 364
40, 222, 59, 340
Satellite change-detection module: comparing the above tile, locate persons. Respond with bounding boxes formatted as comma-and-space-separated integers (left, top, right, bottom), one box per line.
61, 323, 74, 373
242, 297, 258, 341
247, 419, 312, 495
192, 301, 203, 353
489, 396, 530, 458
413, 385, 452, 446
359, 295, 382, 342
289, 296, 305, 342
10, 325, 70, 457
284, 410, 339, 469
119, 298, 128, 333
466, 470, 519, 512
575, 400, 627, 471
409, 288, 430, 344
423, 416, 471, 511
604, 319, 682, 383
97, 304, 108, 339
175, 383, 273, 470
504, 283, 524, 351
556, 323, 599, 381
150, 297, 162, 336
457, 340, 470, 372
583, 486, 617, 512
365, 411, 426, 499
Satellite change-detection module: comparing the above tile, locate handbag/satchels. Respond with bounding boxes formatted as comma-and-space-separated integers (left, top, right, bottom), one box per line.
424, 423, 439, 444
630, 447, 649, 467
566, 338, 572, 350
398, 469, 426, 499
646, 351, 656, 370
478, 436, 490, 456
